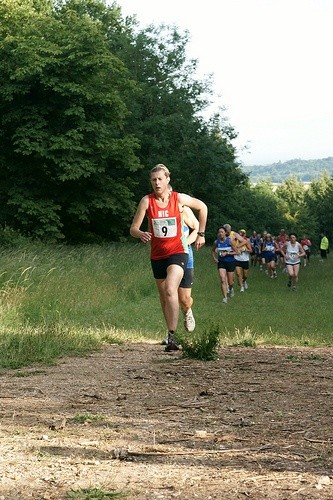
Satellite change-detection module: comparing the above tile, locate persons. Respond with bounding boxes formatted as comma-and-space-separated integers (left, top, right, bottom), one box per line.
212, 227, 238, 304
130, 164, 208, 350
215, 224, 247, 294
250, 228, 313, 278
234, 229, 253, 292
280, 233, 306, 290
167, 184, 200, 332
320, 233, 329, 260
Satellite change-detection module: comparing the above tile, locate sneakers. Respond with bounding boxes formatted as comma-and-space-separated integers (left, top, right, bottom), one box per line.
240, 287, 244, 293
181, 308, 195, 333
229, 288, 233, 297
161, 337, 168, 345
244, 282, 248, 289
222, 298, 227, 303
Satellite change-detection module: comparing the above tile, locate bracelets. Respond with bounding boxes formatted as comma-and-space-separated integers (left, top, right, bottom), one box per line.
227, 252, 230, 254
197, 231, 206, 236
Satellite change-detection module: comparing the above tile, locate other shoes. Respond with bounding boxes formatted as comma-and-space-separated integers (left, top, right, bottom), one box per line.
273, 270, 277, 278
288, 281, 291, 287
270, 271, 273, 279
167, 338, 178, 349
